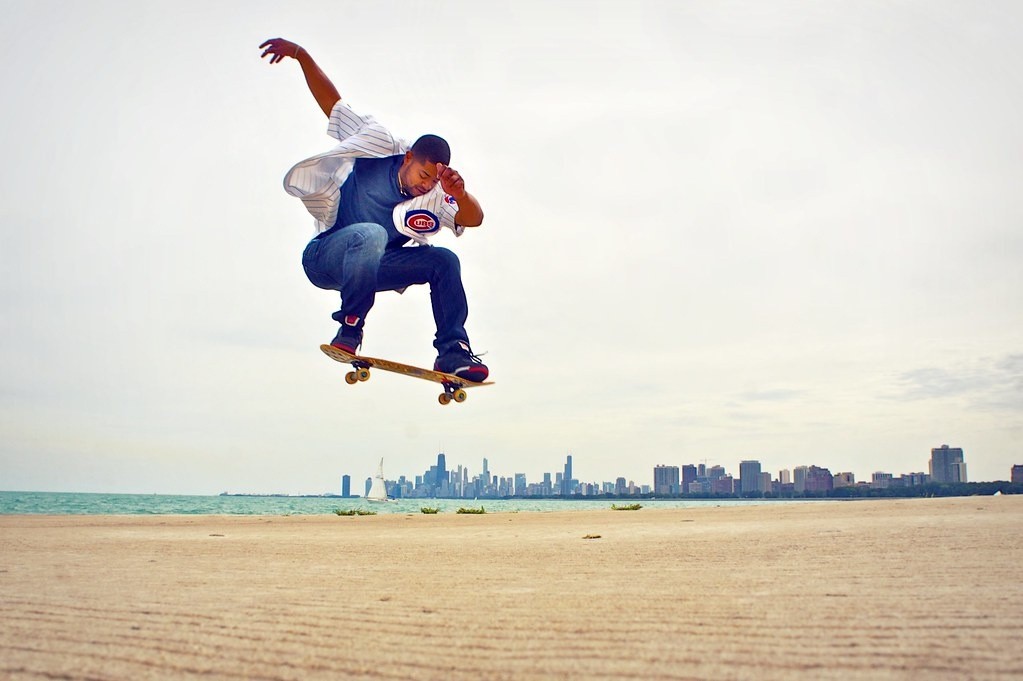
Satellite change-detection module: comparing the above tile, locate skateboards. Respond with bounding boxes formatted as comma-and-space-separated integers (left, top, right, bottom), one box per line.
319, 343, 496, 406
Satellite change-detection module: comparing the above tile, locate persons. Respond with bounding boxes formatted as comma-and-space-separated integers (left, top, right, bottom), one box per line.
259, 38, 490, 382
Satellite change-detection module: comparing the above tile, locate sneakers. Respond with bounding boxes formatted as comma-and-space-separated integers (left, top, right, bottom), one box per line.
433, 341, 489, 381
330, 315, 365, 354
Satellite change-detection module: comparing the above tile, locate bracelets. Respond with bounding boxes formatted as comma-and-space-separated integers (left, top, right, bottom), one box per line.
294, 45, 301, 57
